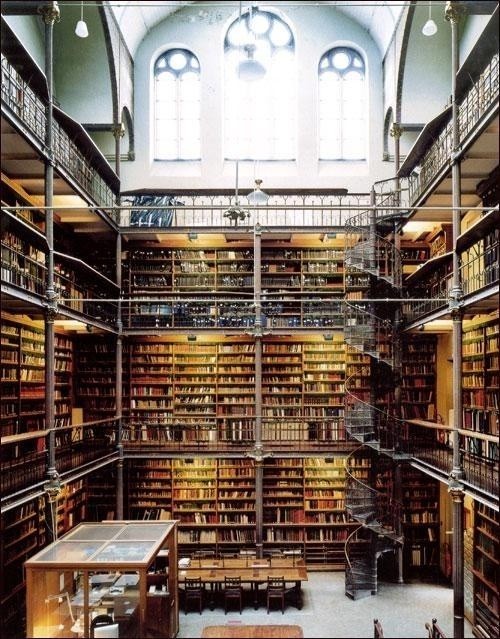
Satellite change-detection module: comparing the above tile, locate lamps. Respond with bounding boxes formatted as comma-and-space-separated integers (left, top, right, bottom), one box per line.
75, 1, 89, 38
422, 1, 438, 36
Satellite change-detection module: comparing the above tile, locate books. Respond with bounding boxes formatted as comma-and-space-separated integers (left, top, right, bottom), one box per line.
1, 193, 93, 316
261, 343, 436, 440
122, 246, 255, 326
259, 245, 431, 326
1, 50, 135, 224
121, 456, 257, 544
406, 52, 499, 208
462, 309, 500, 466
262, 457, 439, 566
2, 450, 117, 600
120, 340, 257, 440
1, 320, 118, 461
472, 503, 499, 615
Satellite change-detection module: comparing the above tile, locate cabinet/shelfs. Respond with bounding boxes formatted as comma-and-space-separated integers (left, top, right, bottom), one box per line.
2, 46, 500, 639
